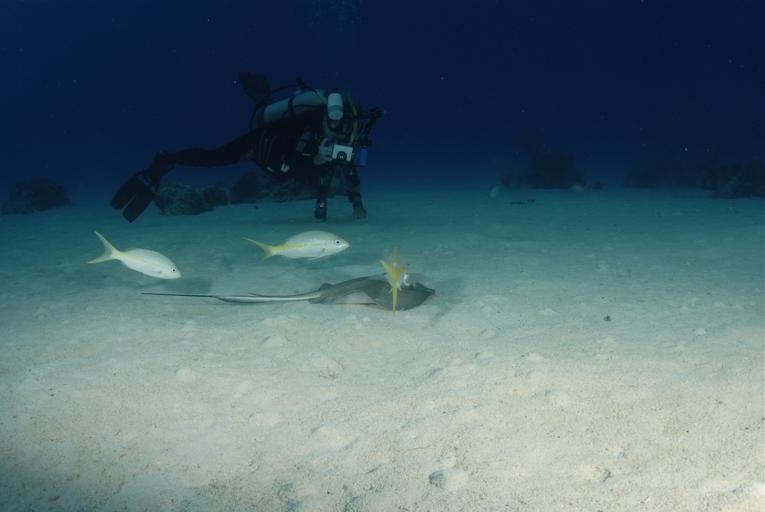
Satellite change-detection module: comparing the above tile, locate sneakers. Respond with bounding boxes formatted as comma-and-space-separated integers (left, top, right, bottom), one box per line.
355, 202, 366, 218
153, 151, 173, 190
315, 198, 326, 219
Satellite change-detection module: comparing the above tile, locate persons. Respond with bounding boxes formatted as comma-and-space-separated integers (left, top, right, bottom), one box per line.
110, 72, 380, 223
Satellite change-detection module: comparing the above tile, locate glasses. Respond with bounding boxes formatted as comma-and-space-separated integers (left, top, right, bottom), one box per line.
328, 119, 351, 136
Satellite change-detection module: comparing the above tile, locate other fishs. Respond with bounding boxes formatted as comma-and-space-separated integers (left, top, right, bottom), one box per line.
141, 272, 435, 312
243, 229, 350, 262
86, 230, 181, 281
